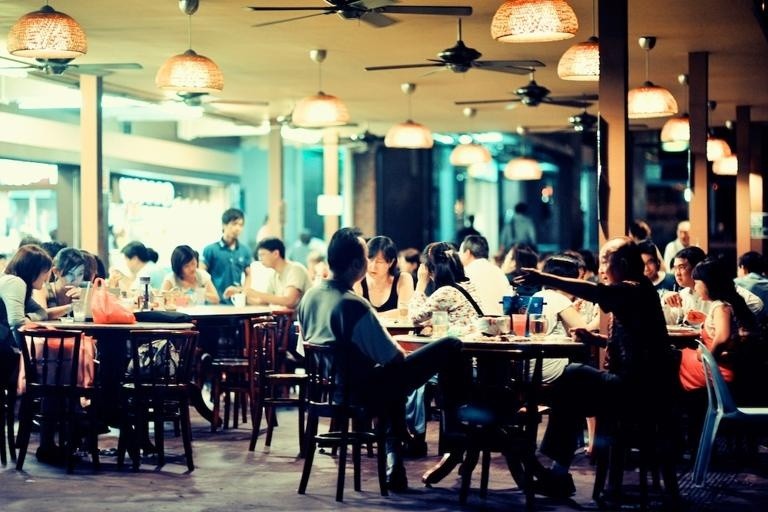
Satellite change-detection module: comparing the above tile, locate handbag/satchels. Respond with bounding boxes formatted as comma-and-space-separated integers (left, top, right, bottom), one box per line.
127, 340, 178, 379
16, 332, 99, 394
92, 277, 136, 323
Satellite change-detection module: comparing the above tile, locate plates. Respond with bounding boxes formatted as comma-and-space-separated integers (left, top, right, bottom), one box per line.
667, 323, 694, 333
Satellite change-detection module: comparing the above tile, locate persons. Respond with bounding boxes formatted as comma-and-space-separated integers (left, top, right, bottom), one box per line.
0, 235, 107, 390
298, 200, 671, 496
203, 206, 312, 309
110, 241, 219, 307
638, 221, 768, 389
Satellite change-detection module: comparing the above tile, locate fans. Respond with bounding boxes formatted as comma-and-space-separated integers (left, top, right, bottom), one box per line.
248, 1, 472, 29
0, 56, 143, 80
366, 17, 546, 75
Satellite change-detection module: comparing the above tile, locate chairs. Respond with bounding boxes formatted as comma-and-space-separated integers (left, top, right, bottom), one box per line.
0, 305, 768, 510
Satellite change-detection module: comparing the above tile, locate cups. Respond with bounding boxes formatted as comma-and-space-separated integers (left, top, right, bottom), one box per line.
231, 293, 246, 306
432, 312, 448, 337
152, 287, 206, 309
512, 314, 527, 337
529, 314, 548, 339
71, 299, 86, 322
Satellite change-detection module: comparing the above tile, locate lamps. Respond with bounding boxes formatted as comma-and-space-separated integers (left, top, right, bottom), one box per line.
293, 49, 353, 127
8, 0, 88, 58
155, 0, 226, 93
384, 81, 489, 168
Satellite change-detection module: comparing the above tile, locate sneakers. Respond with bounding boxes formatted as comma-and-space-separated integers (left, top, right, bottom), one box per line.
405, 440, 427, 461
386, 466, 408, 489
534, 468, 576, 495
34, 445, 83, 466
